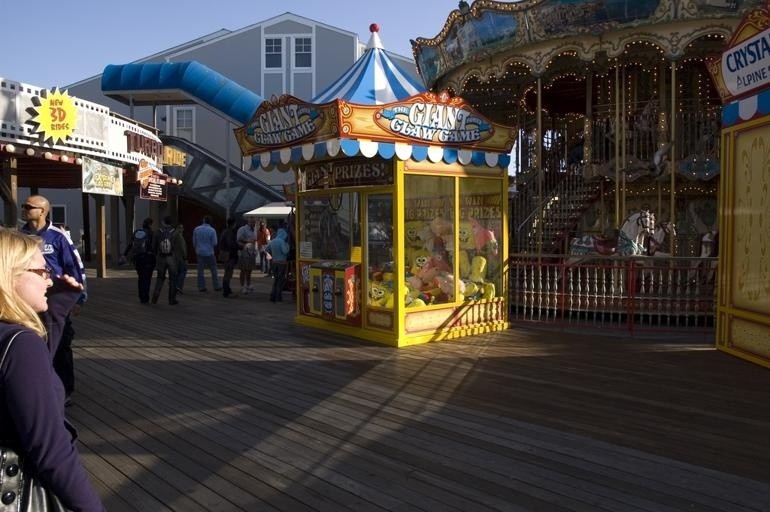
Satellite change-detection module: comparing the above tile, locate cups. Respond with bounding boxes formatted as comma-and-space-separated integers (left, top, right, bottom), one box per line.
25, 267, 53, 279
21, 202, 42, 210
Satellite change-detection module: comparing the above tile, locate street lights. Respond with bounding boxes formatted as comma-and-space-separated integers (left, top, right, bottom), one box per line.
1, 328, 76, 512
218, 250, 230, 263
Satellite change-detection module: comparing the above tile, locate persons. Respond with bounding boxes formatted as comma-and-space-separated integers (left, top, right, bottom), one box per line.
119, 216, 296, 305
20, 194, 88, 408
0, 225, 106, 512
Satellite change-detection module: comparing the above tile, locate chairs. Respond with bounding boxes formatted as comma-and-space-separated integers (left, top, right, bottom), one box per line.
175, 287, 183, 296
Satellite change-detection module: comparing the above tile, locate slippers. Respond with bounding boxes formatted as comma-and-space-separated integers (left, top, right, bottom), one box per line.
157, 226, 175, 256
127, 227, 153, 257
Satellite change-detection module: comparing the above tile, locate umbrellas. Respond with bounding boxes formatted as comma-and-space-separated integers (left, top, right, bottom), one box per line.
169, 300, 178, 305
228, 293, 238, 299
199, 288, 207, 292
